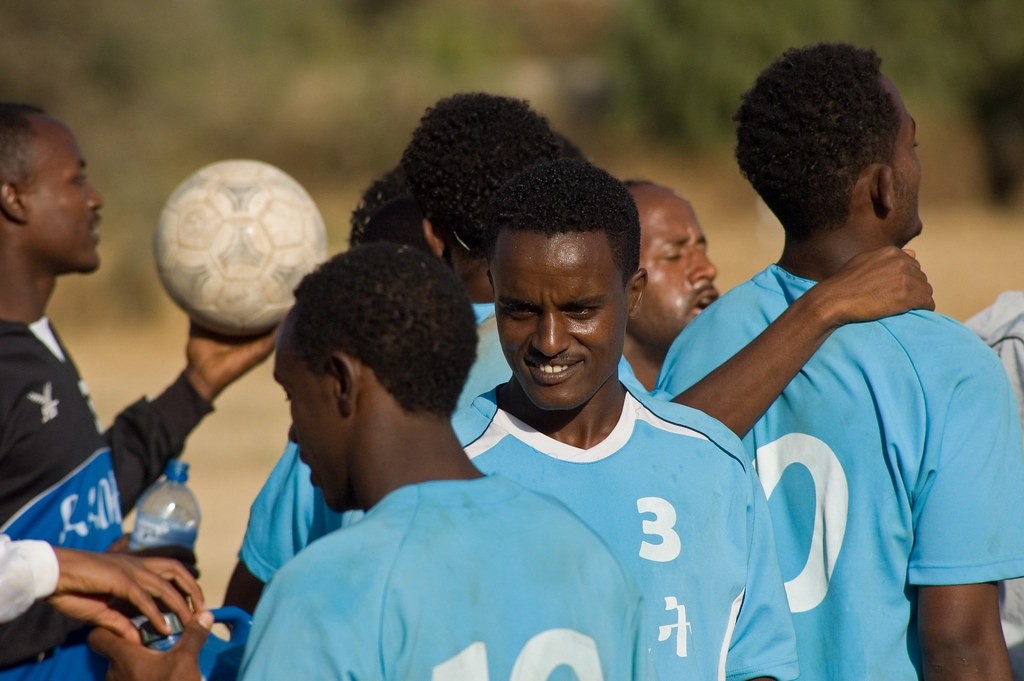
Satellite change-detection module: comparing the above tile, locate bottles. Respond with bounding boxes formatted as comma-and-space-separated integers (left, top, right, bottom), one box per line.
127, 459, 200, 555
138, 607, 254, 681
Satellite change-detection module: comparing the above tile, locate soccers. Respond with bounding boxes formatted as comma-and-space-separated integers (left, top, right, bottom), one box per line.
152, 157, 332, 340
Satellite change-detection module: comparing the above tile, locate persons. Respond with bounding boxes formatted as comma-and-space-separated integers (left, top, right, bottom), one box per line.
87, 243, 632, 681
1, 40, 1022, 681
